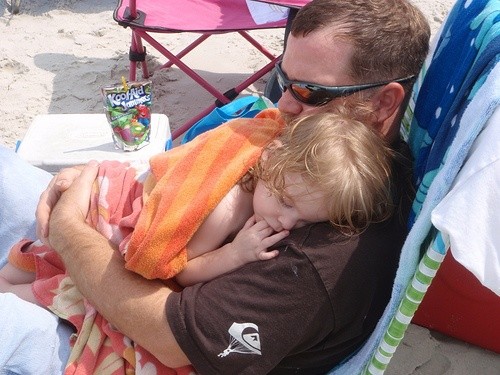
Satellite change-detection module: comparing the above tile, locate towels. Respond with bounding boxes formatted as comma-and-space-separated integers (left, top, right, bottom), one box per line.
326, 0, 500, 375
7, 107, 287, 375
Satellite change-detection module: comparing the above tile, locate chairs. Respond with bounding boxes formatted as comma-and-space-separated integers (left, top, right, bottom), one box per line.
113, 0, 314, 144
159, 0, 500, 375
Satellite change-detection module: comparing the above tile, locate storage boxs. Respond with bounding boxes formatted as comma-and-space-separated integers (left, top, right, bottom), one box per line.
14, 111, 174, 176
